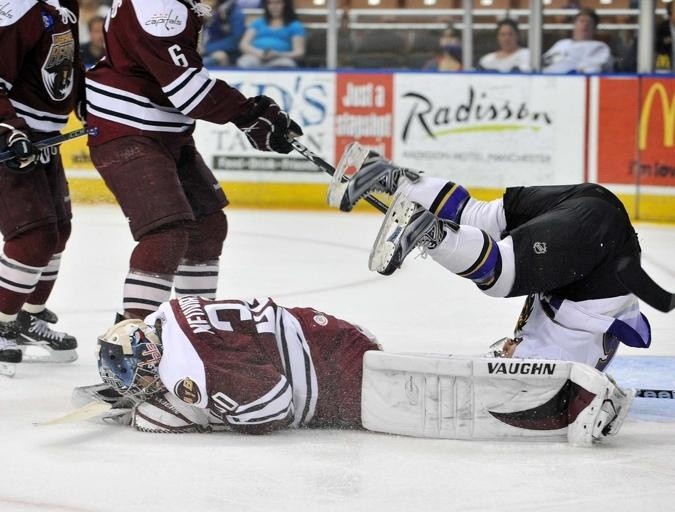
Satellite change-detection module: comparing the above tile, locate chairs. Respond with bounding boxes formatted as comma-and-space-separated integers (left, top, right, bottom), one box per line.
305, 24, 637, 72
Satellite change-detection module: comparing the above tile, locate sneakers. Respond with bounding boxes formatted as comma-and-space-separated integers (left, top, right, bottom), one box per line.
377, 200, 447, 276
338, 149, 402, 213
0, 319, 23, 364
17, 309, 79, 351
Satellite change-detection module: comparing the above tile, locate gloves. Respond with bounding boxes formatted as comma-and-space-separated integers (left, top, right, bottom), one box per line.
3, 129, 42, 174
235, 93, 304, 155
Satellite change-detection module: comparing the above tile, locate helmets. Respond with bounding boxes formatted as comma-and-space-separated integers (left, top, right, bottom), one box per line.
97, 317, 165, 396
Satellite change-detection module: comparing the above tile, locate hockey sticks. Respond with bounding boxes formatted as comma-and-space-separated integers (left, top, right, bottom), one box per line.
33, 400, 112, 428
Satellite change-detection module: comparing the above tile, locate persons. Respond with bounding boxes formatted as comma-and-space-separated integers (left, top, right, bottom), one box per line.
79, 17, 107, 67
1, 0, 86, 363
79, 0, 110, 44
421, 1, 675, 73
237, 1, 308, 70
329, 139, 675, 446
86, 1, 303, 322
197, 1, 245, 66
97, 297, 379, 436
